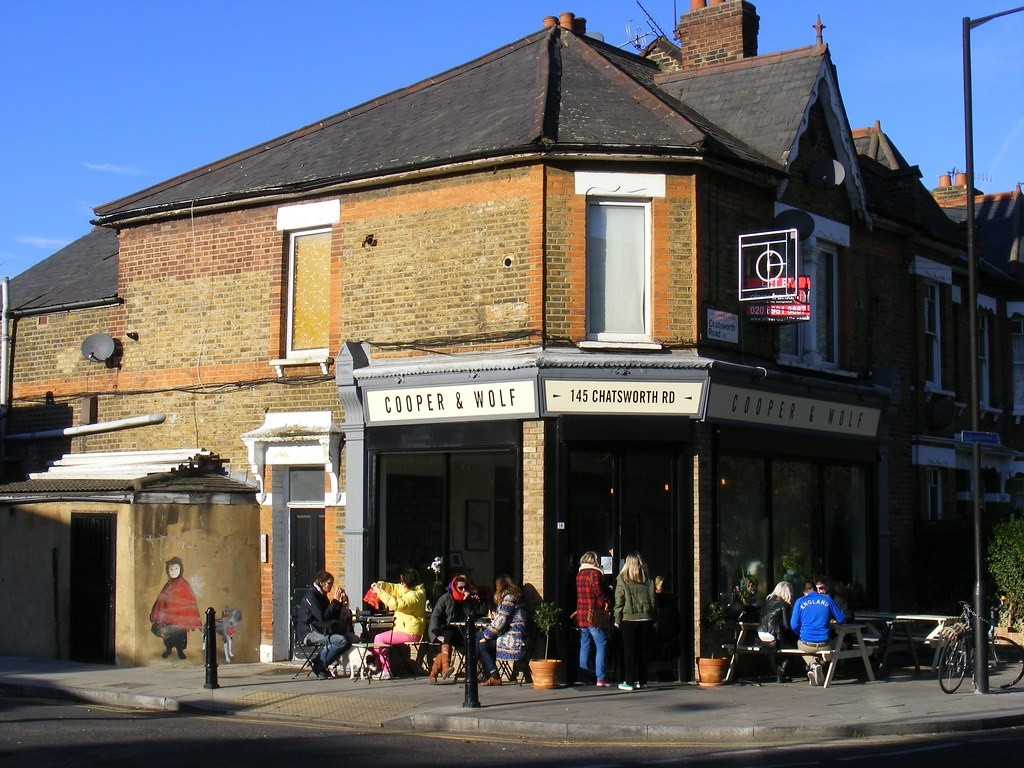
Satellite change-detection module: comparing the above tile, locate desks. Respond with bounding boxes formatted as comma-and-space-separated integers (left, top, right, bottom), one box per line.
858, 619, 920, 677
855, 614, 962, 671
723, 621, 874, 689
450, 623, 489, 639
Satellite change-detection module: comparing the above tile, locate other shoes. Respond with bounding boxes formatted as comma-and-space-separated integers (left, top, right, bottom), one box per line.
813, 664, 825, 686
640, 683, 648, 689
311, 658, 329, 679
808, 670, 818, 685
618, 683, 634, 690
327, 664, 337, 677
624, 681, 641, 689
372, 671, 393, 680
596, 680, 613, 687
480, 676, 502, 686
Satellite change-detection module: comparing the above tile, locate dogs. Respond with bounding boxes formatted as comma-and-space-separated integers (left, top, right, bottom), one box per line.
328, 647, 378, 680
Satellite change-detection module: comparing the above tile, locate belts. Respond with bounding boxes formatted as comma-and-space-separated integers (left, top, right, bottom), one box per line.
801, 639, 828, 647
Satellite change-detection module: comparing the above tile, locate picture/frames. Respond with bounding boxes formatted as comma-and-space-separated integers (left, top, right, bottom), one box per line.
622, 515, 641, 553
465, 500, 491, 551
451, 552, 463, 568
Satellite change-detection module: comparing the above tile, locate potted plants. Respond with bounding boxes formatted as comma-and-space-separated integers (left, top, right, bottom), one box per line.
529, 601, 564, 689
696, 602, 728, 687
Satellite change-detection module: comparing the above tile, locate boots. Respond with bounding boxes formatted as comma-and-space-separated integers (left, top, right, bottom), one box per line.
428, 653, 442, 685
776, 661, 794, 683
441, 644, 454, 680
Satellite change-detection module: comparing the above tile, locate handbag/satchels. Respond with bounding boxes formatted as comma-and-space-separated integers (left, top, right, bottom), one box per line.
363, 587, 381, 611
587, 608, 609, 628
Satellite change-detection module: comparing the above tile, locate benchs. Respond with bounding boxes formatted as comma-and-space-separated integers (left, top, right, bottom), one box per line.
722, 632, 932, 653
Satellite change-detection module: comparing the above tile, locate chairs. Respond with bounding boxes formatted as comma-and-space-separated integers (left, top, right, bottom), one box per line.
291, 615, 538, 687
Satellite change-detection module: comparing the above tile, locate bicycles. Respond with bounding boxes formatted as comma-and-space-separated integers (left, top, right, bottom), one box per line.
937, 598, 1024, 695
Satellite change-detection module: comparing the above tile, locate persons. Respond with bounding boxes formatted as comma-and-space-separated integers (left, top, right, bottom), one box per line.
613, 551, 666, 690
296, 571, 355, 680
477, 574, 530, 686
427, 574, 488, 682
572, 551, 619, 687
371, 569, 427, 682
814, 580, 852, 677
754, 581, 796, 683
789, 581, 846, 686
843, 580, 885, 631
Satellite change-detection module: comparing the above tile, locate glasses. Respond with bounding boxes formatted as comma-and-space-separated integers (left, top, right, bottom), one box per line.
401, 580, 405, 583
456, 586, 466, 591
326, 582, 332, 586
